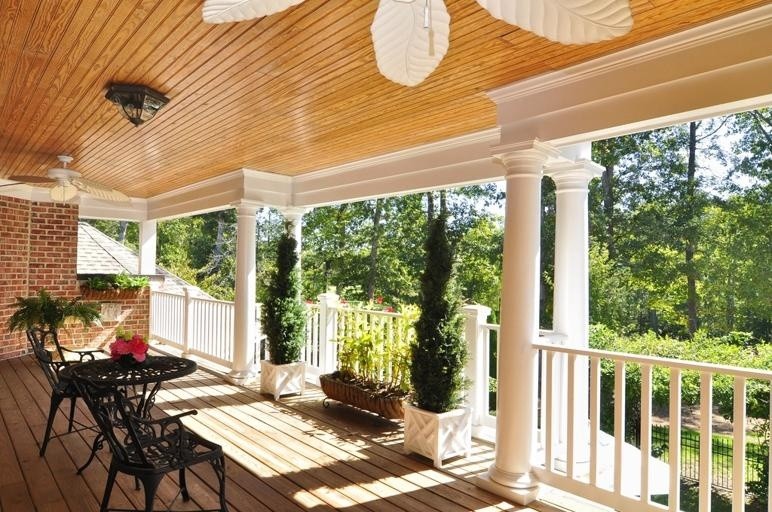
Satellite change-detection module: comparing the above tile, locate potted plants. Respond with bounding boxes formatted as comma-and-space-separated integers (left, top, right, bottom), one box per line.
402, 206, 475, 472
319, 322, 412, 436
259, 218, 317, 402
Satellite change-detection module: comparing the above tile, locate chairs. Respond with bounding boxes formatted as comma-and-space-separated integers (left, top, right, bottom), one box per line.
24, 326, 226, 512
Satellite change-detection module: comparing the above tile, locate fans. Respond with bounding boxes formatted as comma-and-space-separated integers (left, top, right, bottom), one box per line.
200, 0, 636, 89
0, 154, 133, 207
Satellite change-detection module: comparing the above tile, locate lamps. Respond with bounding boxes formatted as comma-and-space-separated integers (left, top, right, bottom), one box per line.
103, 85, 171, 128
